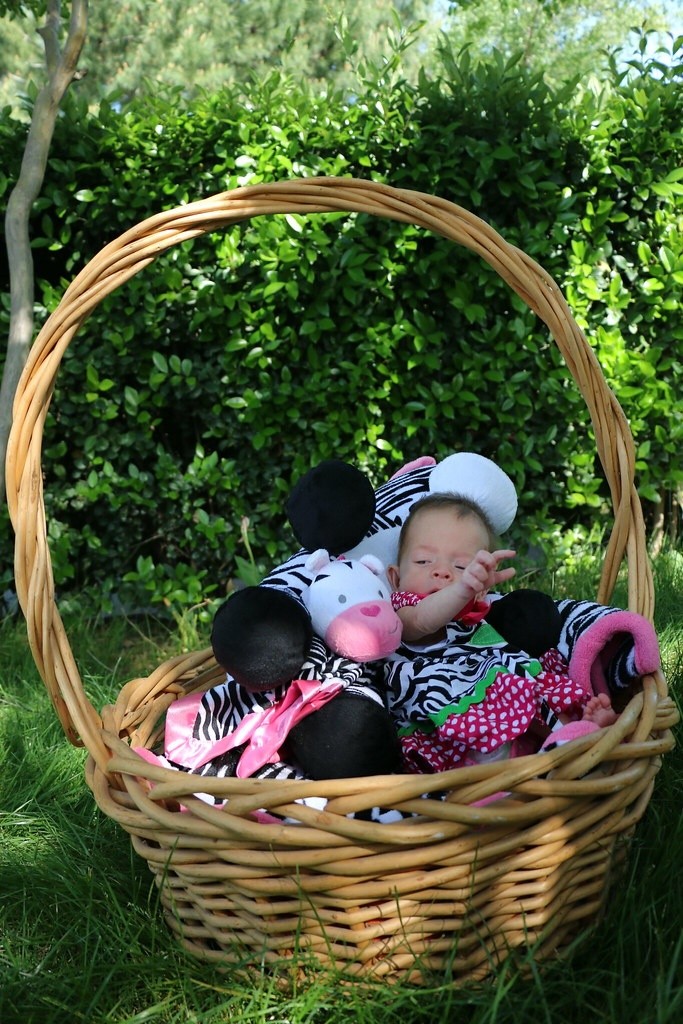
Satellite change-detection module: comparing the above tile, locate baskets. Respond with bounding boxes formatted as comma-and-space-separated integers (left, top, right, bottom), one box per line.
5, 176, 681, 1007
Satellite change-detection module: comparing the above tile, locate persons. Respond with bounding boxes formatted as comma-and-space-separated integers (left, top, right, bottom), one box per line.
392, 491, 616, 784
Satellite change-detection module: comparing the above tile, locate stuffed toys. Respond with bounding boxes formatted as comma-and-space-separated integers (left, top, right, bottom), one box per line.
208, 451, 561, 780
